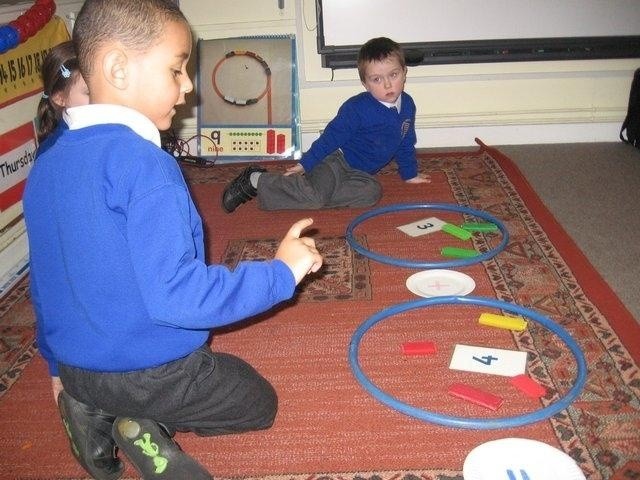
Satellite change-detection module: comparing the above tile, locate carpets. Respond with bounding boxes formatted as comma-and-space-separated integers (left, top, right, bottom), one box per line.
0, 139, 639, 480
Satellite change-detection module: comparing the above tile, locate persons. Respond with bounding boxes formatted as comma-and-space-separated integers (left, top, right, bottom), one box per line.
23, 0, 323, 478
220, 38, 432, 214
37, 41, 90, 146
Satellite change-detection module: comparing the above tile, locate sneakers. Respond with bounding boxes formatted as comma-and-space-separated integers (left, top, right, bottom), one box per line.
57, 388, 125, 480
111, 416, 214, 480
221, 165, 267, 213
